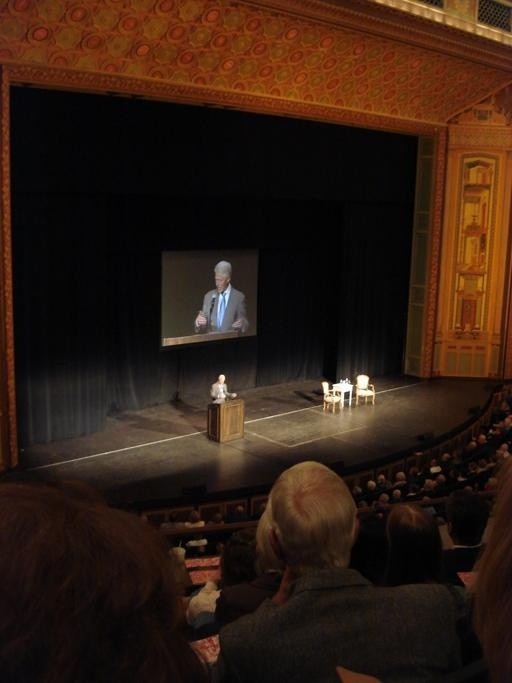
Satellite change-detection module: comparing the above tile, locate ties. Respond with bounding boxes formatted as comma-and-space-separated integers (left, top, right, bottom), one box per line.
217, 292, 227, 330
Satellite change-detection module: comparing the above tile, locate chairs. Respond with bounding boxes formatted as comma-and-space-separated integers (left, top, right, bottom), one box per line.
322, 374, 376, 413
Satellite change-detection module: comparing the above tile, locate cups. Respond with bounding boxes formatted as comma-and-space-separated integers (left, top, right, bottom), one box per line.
171, 547, 187, 563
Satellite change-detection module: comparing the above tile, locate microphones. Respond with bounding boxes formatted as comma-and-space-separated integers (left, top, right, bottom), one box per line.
209, 294, 215, 332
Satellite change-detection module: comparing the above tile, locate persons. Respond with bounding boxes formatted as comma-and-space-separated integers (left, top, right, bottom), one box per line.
193, 261, 249, 334
210, 374, 238, 404
1, 383, 511, 683
